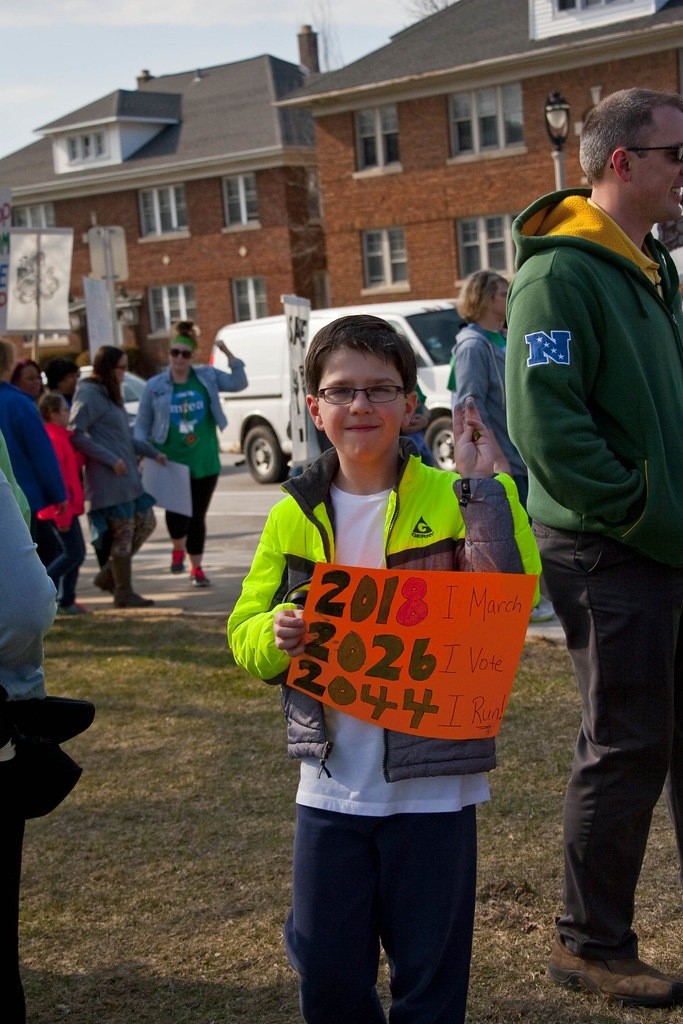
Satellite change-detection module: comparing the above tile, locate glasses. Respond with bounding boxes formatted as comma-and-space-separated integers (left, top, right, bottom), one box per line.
610, 141, 683, 170
317, 383, 410, 404
171, 348, 194, 358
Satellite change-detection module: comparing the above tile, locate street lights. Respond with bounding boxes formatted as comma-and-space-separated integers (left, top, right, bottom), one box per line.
545, 92, 570, 192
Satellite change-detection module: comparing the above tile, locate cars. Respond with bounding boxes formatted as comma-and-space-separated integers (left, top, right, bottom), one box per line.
39, 367, 146, 427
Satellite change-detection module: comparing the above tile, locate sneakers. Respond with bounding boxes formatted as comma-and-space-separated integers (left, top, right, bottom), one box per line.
60, 602, 89, 615
192, 566, 211, 591
546, 936, 683, 1006
170, 552, 188, 573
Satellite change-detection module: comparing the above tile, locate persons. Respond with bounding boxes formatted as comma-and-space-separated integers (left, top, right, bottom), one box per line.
226, 315, 542, 1024
448, 271, 554, 622
133, 320, 248, 587
0, 468, 58, 1024
0, 340, 167, 618
505, 88, 683, 1000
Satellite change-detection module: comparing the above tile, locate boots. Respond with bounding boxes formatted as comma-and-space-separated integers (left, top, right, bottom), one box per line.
96, 560, 112, 596
110, 555, 156, 610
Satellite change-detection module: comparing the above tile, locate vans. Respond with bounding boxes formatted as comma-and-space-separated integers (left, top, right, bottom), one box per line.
211, 301, 468, 484
